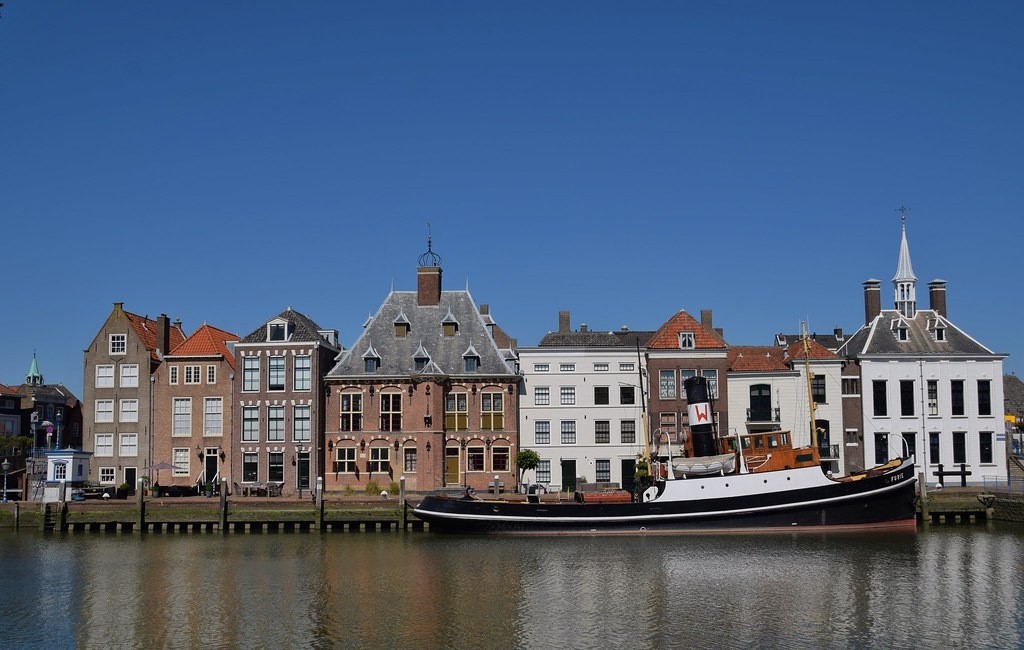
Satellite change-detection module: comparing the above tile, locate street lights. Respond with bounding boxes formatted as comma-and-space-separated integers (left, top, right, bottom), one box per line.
2, 459, 10, 504
56, 411, 61, 450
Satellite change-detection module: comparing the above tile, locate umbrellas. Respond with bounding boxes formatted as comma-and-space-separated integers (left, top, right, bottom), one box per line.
141, 461, 188, 487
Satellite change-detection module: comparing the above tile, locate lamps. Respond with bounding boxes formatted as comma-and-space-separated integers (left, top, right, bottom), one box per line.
471, 384, 477, 395
368, 385, 375, 396
195, 445, 201, 456
425, 440, 431, 451
508, 384, 513, 394
217, 444, 222, 455
326, 386, 332, 397
461, 438, 466, 450
360, 438, 366, 451
291, 456, 296, 466
394, 439, 399, 451
425, 384, 430, 396
486, 437, 491, 450
327, 439, 333, 451
408, 385, 413, 396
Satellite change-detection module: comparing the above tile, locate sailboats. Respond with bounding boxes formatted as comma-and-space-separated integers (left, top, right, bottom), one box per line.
412, 325, 917, 536
